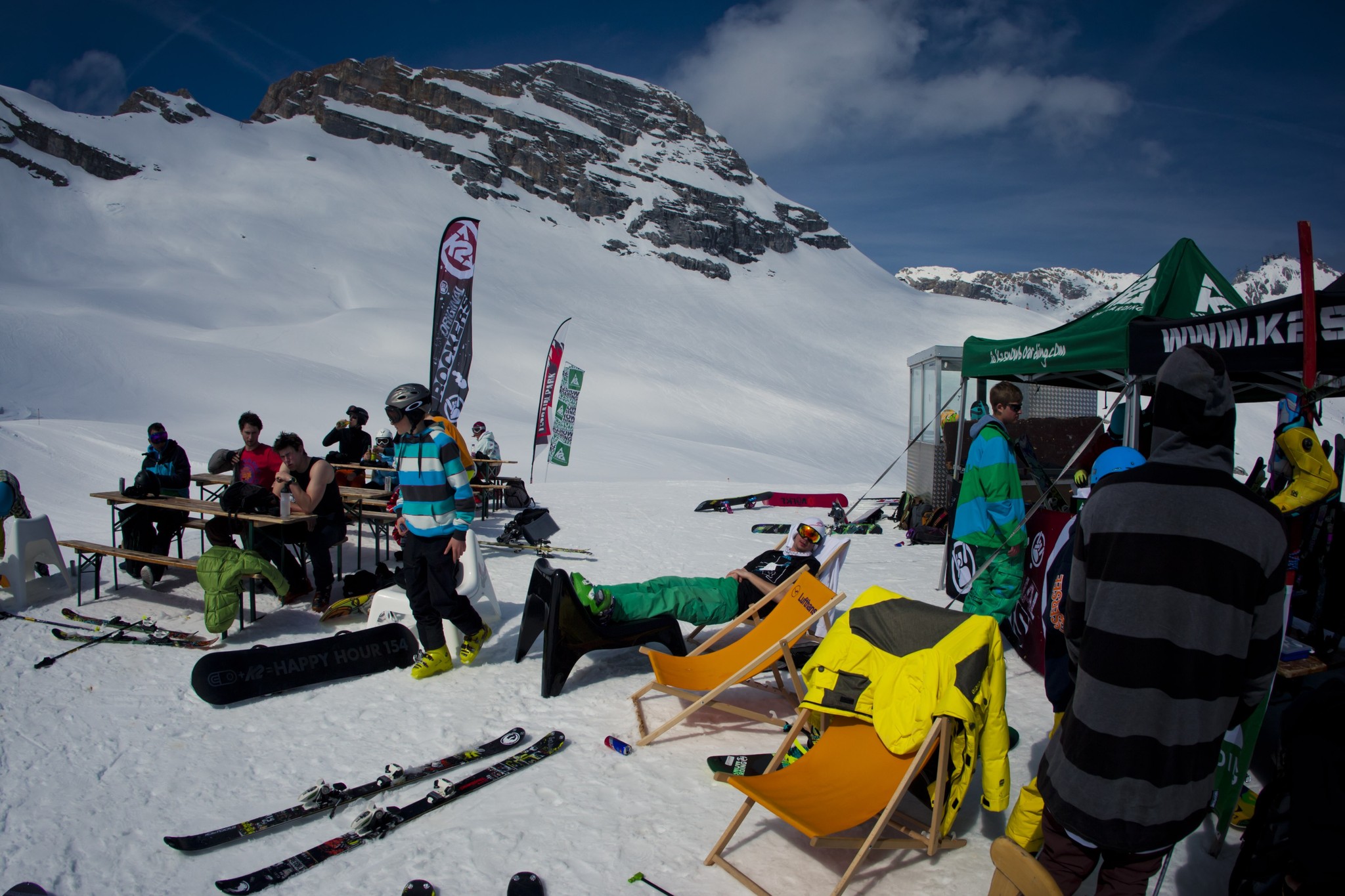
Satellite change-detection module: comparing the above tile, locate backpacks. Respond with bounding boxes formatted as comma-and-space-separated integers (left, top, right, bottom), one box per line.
898, 491, 949, 544
504, 480, 531, 508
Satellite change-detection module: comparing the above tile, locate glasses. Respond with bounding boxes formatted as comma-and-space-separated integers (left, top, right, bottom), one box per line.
994, 403, 1023, 412
349, 415, 358, 419
472, 426, 485, 433
797, 523, 822, 544
385, 407, 405, 423
147, 431, 168, 444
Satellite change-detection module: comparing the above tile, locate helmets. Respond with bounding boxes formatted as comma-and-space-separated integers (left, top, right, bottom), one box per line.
385, 383, 432, 428
792, 517, 826, 551
349, 407, 368, 426
134, 470, 161, 498
1090, 446, 1147, 486
473, 421, 486, 434
375, 428, 393, 443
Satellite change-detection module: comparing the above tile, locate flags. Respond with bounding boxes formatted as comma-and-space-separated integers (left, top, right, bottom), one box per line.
429, 215, 480, 427
533, 316, 573, 447
546, 361, 585, 468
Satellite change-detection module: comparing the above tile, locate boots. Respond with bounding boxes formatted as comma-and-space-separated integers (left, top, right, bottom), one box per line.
1267, 414, 1340, 519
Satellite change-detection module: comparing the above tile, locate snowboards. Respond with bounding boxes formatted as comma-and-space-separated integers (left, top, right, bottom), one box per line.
828, 497, 848, 524
848, 504, 890, 525
762, 491, 849, 507
188, 622, 420, 705
694, 491, 773, 513
706, 746, 808, 777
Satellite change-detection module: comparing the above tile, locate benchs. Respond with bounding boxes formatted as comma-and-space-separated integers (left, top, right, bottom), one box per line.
56, 452, 518, 658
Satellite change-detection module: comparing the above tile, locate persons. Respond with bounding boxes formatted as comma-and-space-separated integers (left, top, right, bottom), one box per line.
118, 421, 192, 590
0, 469, 51, 587
950, 378, 1033, 624
566, 514, 827, 628
1034, 340, 1293, 896
271, 431, 349, 613
321, 406, 372, 477
204, 412, 285, 594
469, 420, 503, 499
995, 445, 1148, 856
360, 427, 402, 528
424, 408, 476, 484
384, 382, 494, 681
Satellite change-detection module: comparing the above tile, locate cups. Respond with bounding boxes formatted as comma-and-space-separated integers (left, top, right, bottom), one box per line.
119, 478, 125, 494
385, 476, 390, 492
280, 483, 290, 520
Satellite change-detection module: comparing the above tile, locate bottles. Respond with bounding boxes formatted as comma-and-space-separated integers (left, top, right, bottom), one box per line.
337, 421, 350, 428
368, 445, 378, 461
471, 444, 476, 459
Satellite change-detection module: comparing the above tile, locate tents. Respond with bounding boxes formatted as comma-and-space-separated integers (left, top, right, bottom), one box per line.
1116, 274, 1344, 473
936, 236, 1259, 601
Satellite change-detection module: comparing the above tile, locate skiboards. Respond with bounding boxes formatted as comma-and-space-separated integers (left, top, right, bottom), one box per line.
477, 539, 594, 558
751, 523, 883, 534
51, 608, 219, 648
163, 725, 565, 895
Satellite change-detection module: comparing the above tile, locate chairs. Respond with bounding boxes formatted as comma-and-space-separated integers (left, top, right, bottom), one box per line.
683, 526, 853, 659
631, 563, 847, 752
704, 581, 1010, 895
513, 558, 689, 699
0, 514, 73, 611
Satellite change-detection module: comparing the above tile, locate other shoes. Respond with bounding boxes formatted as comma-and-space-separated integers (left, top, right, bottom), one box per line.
569, 571, 616, 622
346, 517, 352, 525
141, 565, 156, 589
255, 578, 332, 612
476, 503, 482, 510
411, 643, 453, 678
118, 561, 127, 573
459, 621, 493, 665
